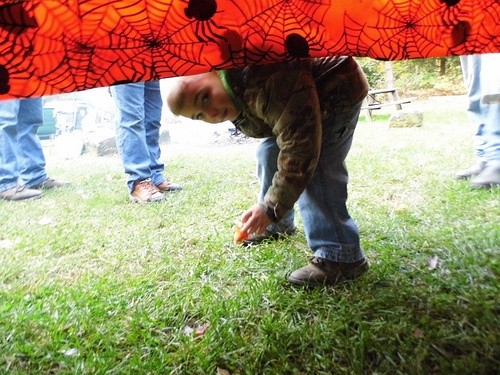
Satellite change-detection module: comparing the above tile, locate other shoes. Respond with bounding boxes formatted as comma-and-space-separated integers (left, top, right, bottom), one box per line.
156, 178, 182, 191
129, 178, 165, 204
288, 256, 367, 288
0, 185, 44, 201
31, 177, 70, 189
243, 227, 296, 246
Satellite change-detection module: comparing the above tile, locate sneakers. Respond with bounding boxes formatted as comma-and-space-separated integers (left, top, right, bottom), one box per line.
470, 160, 500, 184
456, 159, 485, 179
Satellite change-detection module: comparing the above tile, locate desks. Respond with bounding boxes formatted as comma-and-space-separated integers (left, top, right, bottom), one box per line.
367, 87, 402, 119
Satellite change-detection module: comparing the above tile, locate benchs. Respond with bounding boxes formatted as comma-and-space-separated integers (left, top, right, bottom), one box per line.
360, 97, 411, 122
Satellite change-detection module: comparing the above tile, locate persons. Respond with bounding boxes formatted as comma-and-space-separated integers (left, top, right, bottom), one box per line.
159, 55, 371, 288
108, 79, 183, 203
456, 54, 500, 187
0, 97, 65, 201
